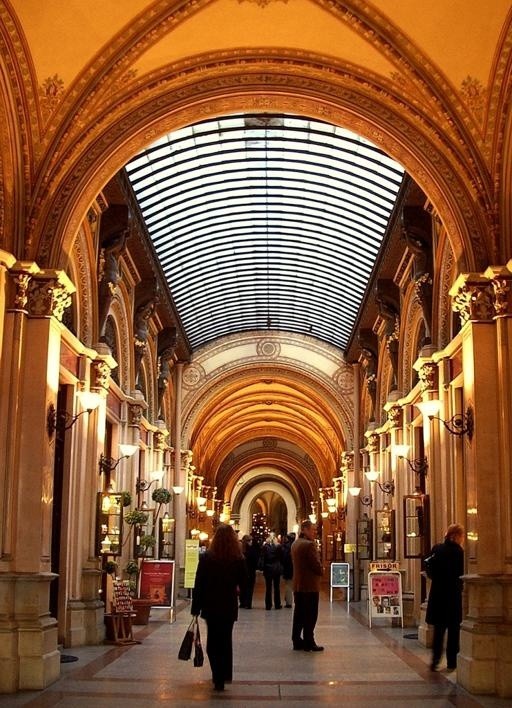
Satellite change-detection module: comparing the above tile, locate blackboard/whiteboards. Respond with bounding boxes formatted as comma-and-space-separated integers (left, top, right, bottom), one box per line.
330, 562, 350, 587
368, 571, 403, 618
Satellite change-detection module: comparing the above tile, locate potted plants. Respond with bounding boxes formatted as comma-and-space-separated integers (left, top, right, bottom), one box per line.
102, 487, 172, 639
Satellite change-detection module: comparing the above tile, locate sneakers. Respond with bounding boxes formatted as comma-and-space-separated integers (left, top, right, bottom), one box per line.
239, 605, 292, 610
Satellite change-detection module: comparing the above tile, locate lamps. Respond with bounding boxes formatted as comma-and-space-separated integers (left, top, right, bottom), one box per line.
365, 472, 395, 496
308, 498, 345, 525
391, 445, 427, 476
99, 445, 141, 475
414, 400, 474, 442
170, 487, 214, 522
348, 488, 372, 507
48, 390, 102, 438
135, 472, 162, 494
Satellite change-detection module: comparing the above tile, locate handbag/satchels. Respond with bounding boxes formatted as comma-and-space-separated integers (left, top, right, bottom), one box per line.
194, 644, 204, 667
424, 555, 435, 580
178, 631, 194, 661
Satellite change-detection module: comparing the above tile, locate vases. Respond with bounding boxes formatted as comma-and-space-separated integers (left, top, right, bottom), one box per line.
105, 613, 136, 641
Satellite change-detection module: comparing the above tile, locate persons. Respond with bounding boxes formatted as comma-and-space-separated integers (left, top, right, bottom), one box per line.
291, 520, 324, 652
424, 523, 465, 675
239, 532, 297, 610
178, 525, 244, 692
373, 595, 398, 615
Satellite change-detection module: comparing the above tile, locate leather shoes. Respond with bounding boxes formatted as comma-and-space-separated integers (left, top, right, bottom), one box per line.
294, 644, 324, 652
